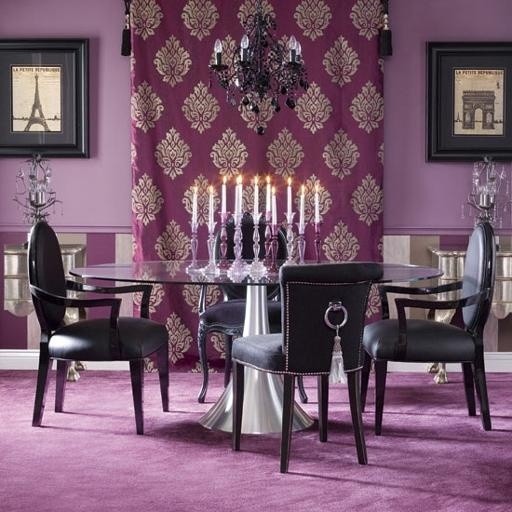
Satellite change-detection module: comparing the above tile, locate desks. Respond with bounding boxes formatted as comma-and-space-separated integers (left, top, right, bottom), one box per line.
0, 243, 88, 384
66, 259, 444, 437
427, 246, 512, 386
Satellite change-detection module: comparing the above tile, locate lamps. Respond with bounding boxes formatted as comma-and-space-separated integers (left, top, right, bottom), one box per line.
458, 152, 512, 252
206, 1, 309, 138
12, 154, 66, 249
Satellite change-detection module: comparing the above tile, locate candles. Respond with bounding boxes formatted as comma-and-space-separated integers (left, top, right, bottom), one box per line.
191, 173, 323, 235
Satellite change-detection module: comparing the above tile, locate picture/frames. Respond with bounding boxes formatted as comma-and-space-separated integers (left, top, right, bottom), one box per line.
424, 39, 512, 163
0, 37, 90, 160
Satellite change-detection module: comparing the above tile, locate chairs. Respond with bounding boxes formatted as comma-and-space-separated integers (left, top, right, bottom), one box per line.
357, 221, 496, 435
197, 217, 309, 404
230, 260, 385, 474
26, 221, 170, 436
